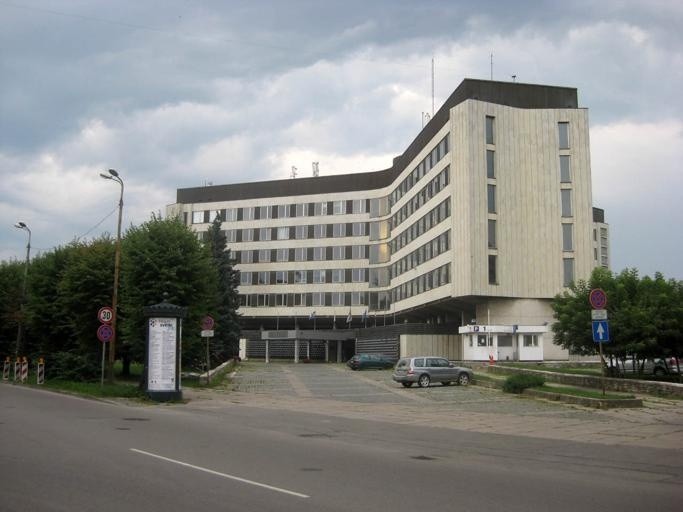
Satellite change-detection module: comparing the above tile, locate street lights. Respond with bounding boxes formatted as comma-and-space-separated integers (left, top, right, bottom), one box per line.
13, 220, 34, 357
98, 167, 127, 386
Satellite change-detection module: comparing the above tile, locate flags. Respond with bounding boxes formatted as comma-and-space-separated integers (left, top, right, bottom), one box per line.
309, 310, 316, 320
345, 310, 352, 323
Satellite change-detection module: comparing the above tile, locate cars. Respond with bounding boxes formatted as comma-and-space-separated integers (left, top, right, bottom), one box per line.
346, 351, 396, 371
391, 355, 474, 389
606, 352, 683, 378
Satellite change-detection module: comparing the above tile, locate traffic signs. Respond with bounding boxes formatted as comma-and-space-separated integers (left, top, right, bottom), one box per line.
97, 307, 115, 324
